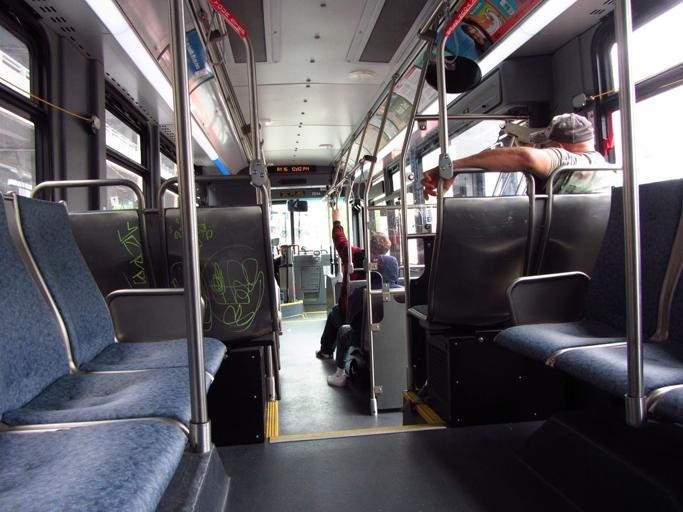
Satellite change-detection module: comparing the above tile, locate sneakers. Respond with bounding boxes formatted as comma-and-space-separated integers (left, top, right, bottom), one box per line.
412, 358, 429, 397
327, 367, 346, 388
316, 350, 334, 360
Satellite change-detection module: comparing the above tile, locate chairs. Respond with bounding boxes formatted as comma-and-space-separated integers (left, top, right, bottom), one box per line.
0, 176, 281, 511
335, 267, 366, 305
406, 180, 682, 430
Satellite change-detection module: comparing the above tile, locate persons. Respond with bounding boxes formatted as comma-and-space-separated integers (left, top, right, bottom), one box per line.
309, 206, 391, 360
420, 115, 621, 201
326, 256, 401, 388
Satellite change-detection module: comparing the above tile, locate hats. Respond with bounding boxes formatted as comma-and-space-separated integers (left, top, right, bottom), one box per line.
543, 112, 595, 144
